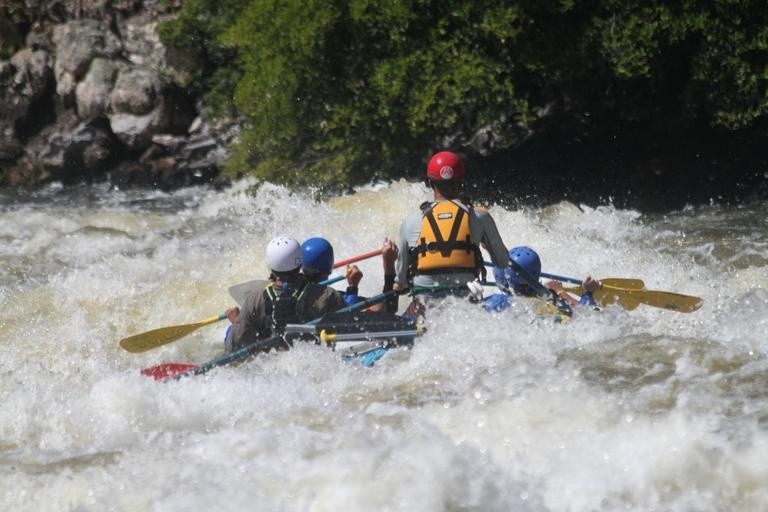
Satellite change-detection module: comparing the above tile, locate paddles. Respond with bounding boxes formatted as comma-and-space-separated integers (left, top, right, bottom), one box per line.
120, 268, 352, 353
229, 249, 383, 306
140, 286, 410, 384
475, 262, 704, 312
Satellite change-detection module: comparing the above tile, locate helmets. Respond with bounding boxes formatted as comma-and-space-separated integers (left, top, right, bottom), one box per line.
493, 246, 542, 294
426, 150, 464, 181
265, 235, 335, 276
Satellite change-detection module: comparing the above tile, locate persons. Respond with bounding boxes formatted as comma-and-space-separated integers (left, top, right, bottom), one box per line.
300, 237, 399, 313
394, 152, 510, 302
222, 237, 362, 353
483, 246, 599, 325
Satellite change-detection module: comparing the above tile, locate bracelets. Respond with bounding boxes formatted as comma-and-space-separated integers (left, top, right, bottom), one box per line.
557, 291, 565, 296
